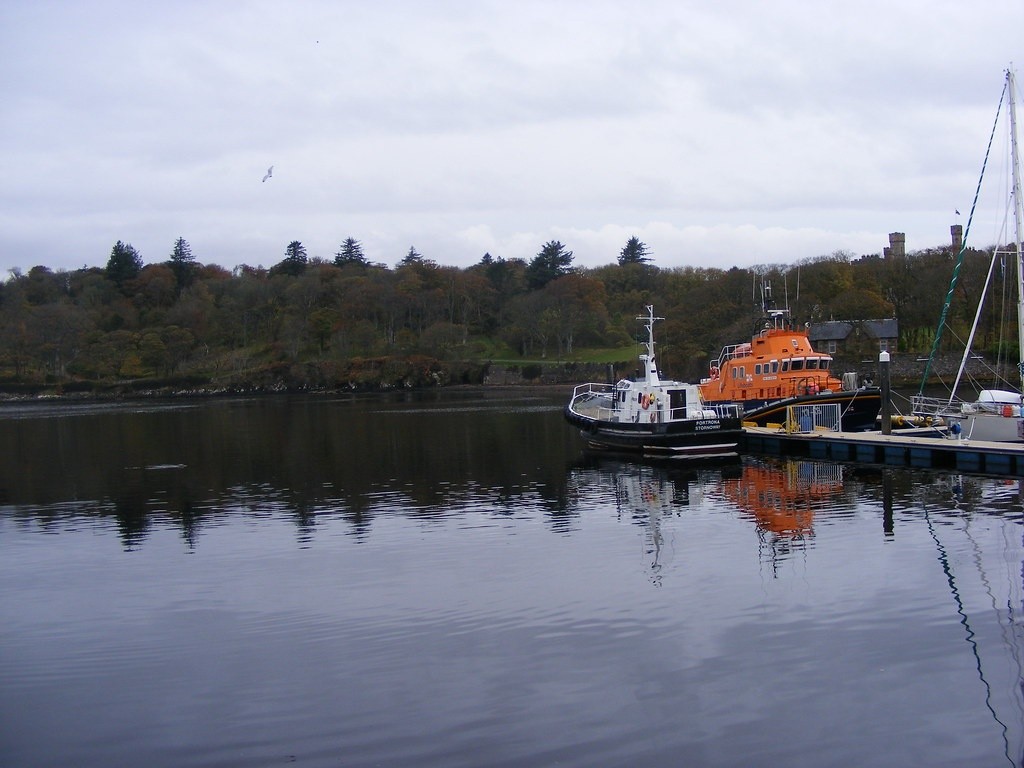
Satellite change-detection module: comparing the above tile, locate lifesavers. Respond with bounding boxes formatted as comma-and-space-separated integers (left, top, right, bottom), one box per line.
640, 393, 650, 410
709, 366, 718, 380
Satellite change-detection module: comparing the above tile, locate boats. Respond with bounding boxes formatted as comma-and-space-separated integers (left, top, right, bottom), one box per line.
564, 305, 746, 464
698, 261, 881, 431
741, 69, 1024, 478
